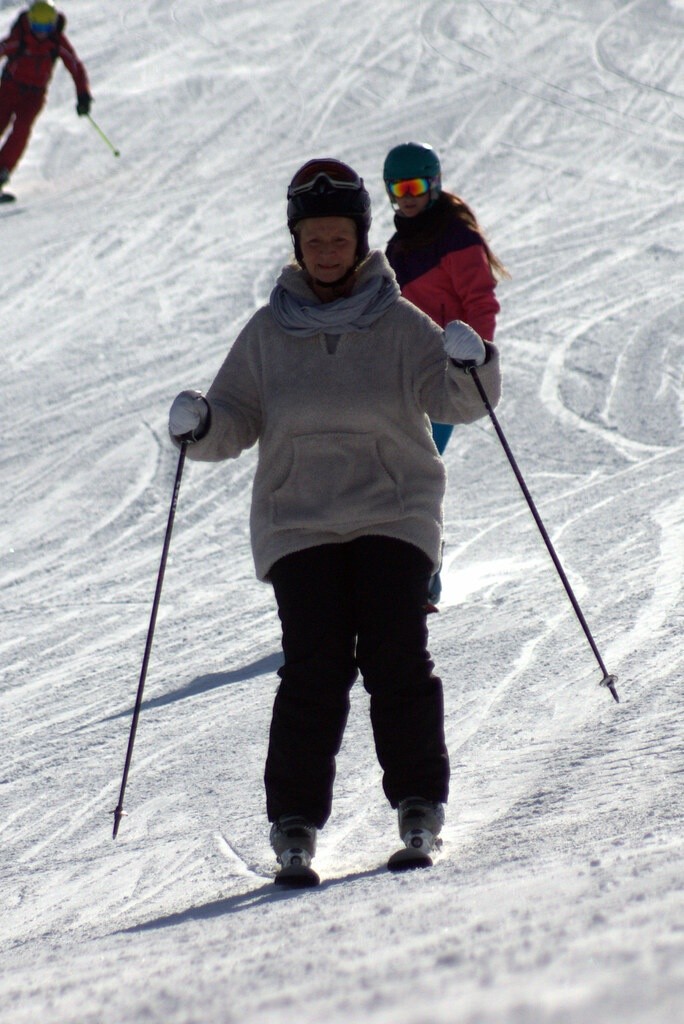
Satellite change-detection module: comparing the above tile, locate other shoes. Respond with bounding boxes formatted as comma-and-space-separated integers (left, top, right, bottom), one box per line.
279, 847, 311, 866
403, 827, 434, 855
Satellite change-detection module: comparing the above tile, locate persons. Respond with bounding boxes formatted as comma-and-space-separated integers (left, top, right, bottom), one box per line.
168, 158, 501, 883
381, 142, 500, 455
0, 0, 93, 204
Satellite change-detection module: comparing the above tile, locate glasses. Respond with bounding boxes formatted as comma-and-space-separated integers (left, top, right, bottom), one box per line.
388, 177, 432, 198
286, 159, 364, 194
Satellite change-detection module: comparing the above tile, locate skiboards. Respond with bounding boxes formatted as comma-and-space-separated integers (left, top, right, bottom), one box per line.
274, 848, 434, 887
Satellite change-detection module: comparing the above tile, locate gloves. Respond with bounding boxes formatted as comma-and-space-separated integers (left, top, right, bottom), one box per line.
75, 94, 90, 114
168, 390, 209, 442
440, 321, 486, 367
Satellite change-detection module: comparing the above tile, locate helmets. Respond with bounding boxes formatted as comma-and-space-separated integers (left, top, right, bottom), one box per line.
29, 0, 58, 32
287, 158, 371, 233
383, 141, 442, 198
